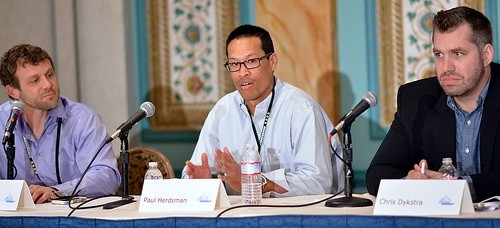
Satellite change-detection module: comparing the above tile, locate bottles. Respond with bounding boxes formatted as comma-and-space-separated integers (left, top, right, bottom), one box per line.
437, 158, 458, 180
144, 162, 163, 180
241, 143, 262, 205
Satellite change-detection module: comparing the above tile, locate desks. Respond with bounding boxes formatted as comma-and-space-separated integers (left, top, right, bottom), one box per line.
0, 196, 500, 228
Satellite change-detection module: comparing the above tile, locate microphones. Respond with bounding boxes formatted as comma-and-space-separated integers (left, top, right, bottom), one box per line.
2, 100, 24, 145
105, 101, 155, 144
330, 91, 377, 135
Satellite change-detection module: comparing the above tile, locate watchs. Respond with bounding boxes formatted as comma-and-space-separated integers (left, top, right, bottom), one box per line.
261, 175, 267, 191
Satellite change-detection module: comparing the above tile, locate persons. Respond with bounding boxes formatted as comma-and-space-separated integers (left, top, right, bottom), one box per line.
365, 5, 500, 202
182, 24, 344, 197
0, 44, 122, 204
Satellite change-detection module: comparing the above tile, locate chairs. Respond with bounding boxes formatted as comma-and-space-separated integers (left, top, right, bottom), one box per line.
115, 147, 174, 196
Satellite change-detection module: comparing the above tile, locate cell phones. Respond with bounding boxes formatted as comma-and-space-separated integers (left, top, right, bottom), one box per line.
52, 196, 86, 205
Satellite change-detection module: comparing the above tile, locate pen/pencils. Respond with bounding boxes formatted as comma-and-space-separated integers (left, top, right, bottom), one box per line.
49, 195, 79, 200
421, 162, 425, 174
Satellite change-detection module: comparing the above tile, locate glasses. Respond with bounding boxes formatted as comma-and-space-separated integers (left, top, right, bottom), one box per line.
223, 52, 274, 72
